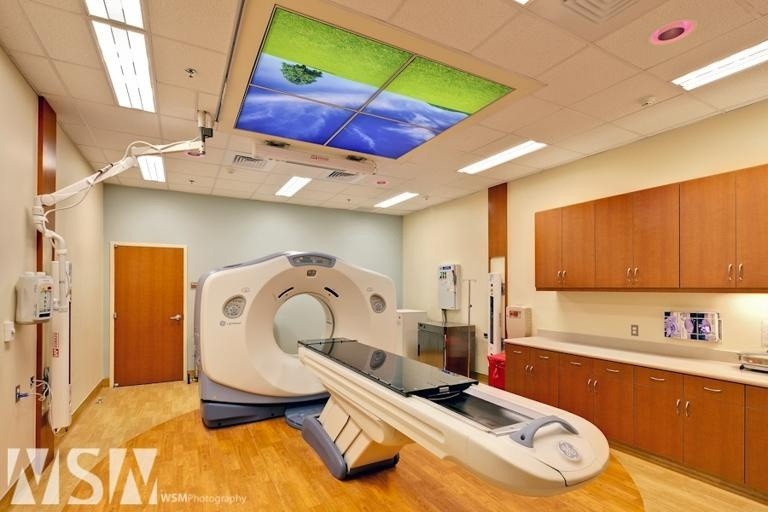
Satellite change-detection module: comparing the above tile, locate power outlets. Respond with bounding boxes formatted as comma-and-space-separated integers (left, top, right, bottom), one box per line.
29, 374, 35, 390
16, 385, 21, 404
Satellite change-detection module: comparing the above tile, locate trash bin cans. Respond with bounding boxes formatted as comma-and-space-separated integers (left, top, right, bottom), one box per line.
486, 352, 506, 391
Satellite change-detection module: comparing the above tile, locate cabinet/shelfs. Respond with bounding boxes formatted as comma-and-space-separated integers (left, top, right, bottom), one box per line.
597, 184, 678, 289
636, 366, 744, 486
560, 353, 634, 447
679, 165, 768, 289
746, 385, 768, 497
504, 343, 558, 407
535, 201, 596, 289
